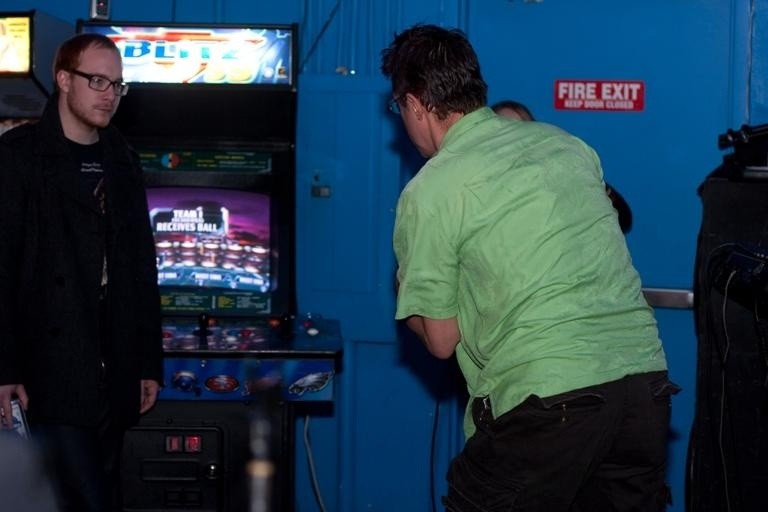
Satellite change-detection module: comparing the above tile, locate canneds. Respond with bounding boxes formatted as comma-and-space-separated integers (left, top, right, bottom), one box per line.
0, 399, 33, 442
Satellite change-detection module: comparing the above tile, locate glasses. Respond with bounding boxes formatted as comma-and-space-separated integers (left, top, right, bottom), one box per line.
387, 96, 409, 114
68, 69, 130, 97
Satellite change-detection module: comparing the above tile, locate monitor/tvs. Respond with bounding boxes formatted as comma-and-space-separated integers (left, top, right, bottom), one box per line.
141, 185, 281, 297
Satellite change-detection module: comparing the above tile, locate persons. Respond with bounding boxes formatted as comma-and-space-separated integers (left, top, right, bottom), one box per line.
377, 19, 683, 512
0, 30, 168, 511
490, 98, 634, 236
